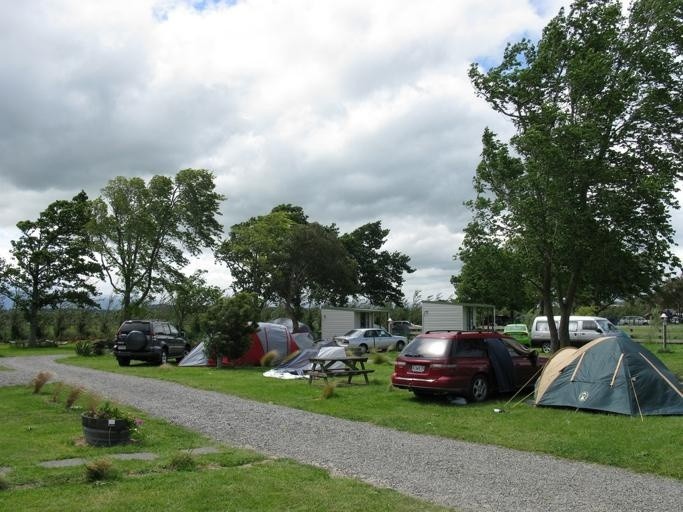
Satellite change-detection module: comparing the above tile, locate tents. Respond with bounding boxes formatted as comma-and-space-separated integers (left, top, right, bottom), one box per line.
264, 337, 360, 379
535, 333, 682, 417
178, 321, 299, 368
272, 317, 314, 347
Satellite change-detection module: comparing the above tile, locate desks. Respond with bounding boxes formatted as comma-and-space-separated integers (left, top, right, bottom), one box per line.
306, 357, 369, 385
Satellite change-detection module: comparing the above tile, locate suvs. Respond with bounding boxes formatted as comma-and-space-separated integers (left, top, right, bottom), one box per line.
109, 319, 192, 368
390, 328, 549, 404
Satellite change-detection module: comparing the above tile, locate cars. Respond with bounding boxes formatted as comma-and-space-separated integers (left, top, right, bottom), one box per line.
391, 320, 422, 330
503, 323, 530, 347
332, 326, 409, 355
617, 315, 651, 325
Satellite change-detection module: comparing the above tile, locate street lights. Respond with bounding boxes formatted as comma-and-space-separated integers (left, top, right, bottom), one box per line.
659, 313, 670, 349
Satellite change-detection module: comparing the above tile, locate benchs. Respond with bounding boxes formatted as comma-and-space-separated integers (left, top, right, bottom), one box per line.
302, 367, 358, 385
314, 369, 375, 385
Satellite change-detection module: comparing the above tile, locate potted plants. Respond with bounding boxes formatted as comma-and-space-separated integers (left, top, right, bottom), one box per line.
80, 399, 133, 447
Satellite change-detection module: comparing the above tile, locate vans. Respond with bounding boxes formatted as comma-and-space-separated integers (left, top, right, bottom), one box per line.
529, 315, 632, 355
